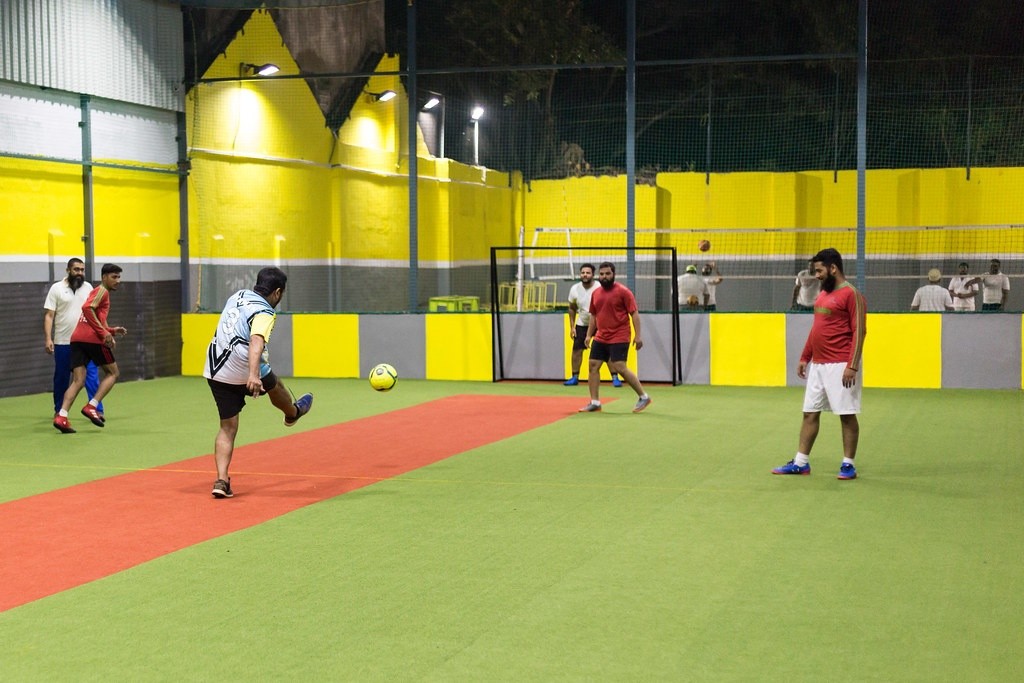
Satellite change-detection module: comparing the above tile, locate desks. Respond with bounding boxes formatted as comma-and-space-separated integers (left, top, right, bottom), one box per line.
428, 295, 481, 311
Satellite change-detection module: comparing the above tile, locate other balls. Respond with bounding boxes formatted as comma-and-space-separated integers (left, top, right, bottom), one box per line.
697, 239, 710, 252
687, 296, 700, 305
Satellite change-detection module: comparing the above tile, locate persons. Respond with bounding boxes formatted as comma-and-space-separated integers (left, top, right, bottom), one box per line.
53, 263, 127, 433
771, 247, 866, 480
948, 262, 979, 311
789, 259, 822, 310
202, 265, 314, 497
578, 261, 651, 413
964, 259, 1010, 311
563, 263, 623, 387
677, 265, 710, 311
44, 258, 106, 422
692, 262, 723, 311
910, 269, 955, 311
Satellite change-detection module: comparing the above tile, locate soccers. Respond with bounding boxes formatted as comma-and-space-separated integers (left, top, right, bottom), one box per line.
369, 364, 398, 392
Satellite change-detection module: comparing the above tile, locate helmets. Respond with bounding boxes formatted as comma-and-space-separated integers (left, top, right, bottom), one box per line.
687, 295, 699, 304
701, 264, 711, 275
928, 268, 941, 283
686, 265, 697, 272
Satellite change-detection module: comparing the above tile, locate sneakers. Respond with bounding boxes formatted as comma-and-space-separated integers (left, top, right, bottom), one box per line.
563, 377, 578, 385
81, 403, 105, 427
53, 414, 76, 433
632, 394, 652, 413
612, 375, 622, 387
283, 392, 314, 426
773, 460, 812, 476
577, 400, 601, 413
212, 477, 234, 497
837, 462, 857, 480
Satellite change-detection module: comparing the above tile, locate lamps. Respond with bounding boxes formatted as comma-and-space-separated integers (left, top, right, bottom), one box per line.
419, 94, 440, 110
239, 61, 280, 76
365, 90, 398, 103
